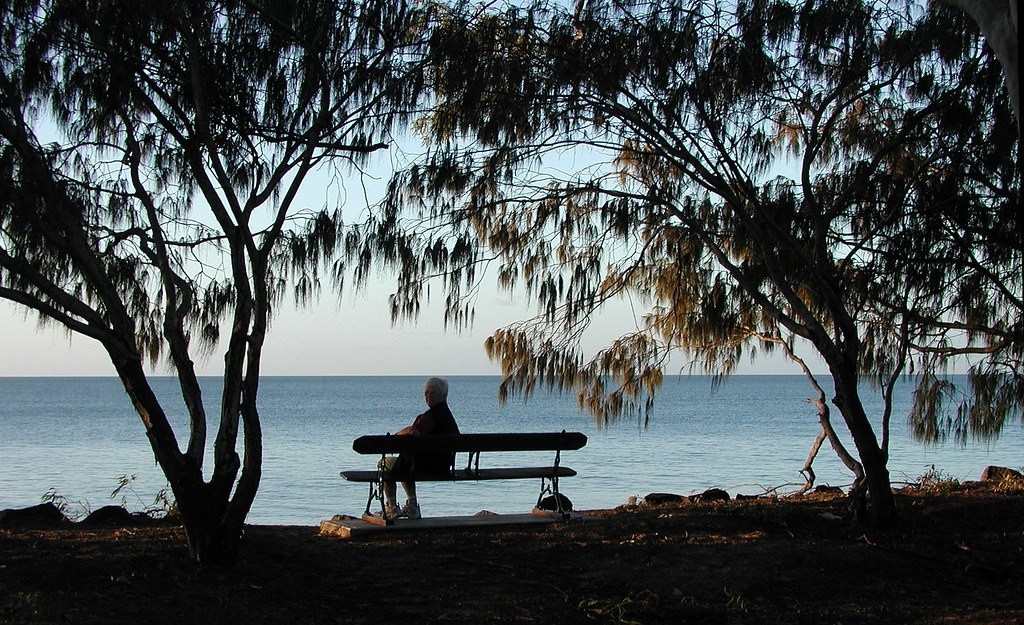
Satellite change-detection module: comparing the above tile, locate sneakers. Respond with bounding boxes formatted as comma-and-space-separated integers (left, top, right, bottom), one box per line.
400, 499, 421, 520
373, 502, 402, 520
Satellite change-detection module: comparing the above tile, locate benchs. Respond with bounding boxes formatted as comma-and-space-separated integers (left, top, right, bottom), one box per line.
339, 430, 587, 526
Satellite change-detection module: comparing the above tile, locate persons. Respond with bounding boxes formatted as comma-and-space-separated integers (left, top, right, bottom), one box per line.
374, 378, 460, 519
